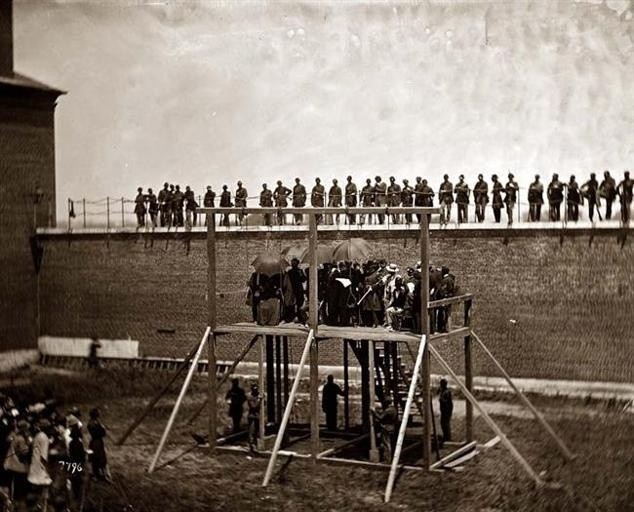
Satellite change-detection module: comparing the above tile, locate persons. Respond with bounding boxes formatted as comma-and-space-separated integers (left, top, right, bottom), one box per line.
260, 176, 307, 226
323, 374, 346, 429
438, 173, 519, 225
435, 379, 454, 441
244, 383, 266, 454
328, 178, 342, 225
203, 181, 248, 226
226, 378, 247, 432
0, 395, 115, 512
567, 174, 579, 222
581, 172, 599, 222
344, 175, 357, 225
134, 181, 197, 228
527, 173, 563, 221
359, 176, 435, 225
311, 177, 325, 225
85, 333, 104, 378
244, 236, 457, 335
368, 396, 399, 465
599, 170, 634, 220
